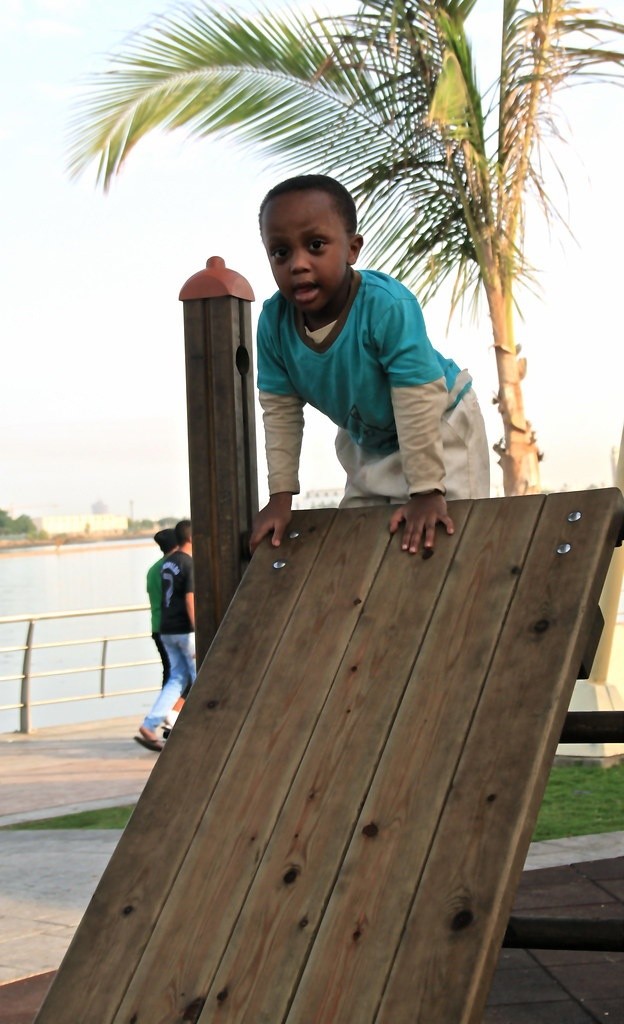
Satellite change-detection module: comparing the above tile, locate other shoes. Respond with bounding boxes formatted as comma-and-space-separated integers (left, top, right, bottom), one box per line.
161, 725, 171, 738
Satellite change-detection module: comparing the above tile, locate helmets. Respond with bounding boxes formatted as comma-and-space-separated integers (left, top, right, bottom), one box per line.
154, 528, 179, 556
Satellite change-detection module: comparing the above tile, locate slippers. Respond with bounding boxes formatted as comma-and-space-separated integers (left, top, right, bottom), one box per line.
133, 727, 163, 752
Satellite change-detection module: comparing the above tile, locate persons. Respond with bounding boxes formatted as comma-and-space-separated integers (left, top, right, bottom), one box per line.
135, 521, 193, 752
248, 174, 492, 556
147, 529, 189, 738
